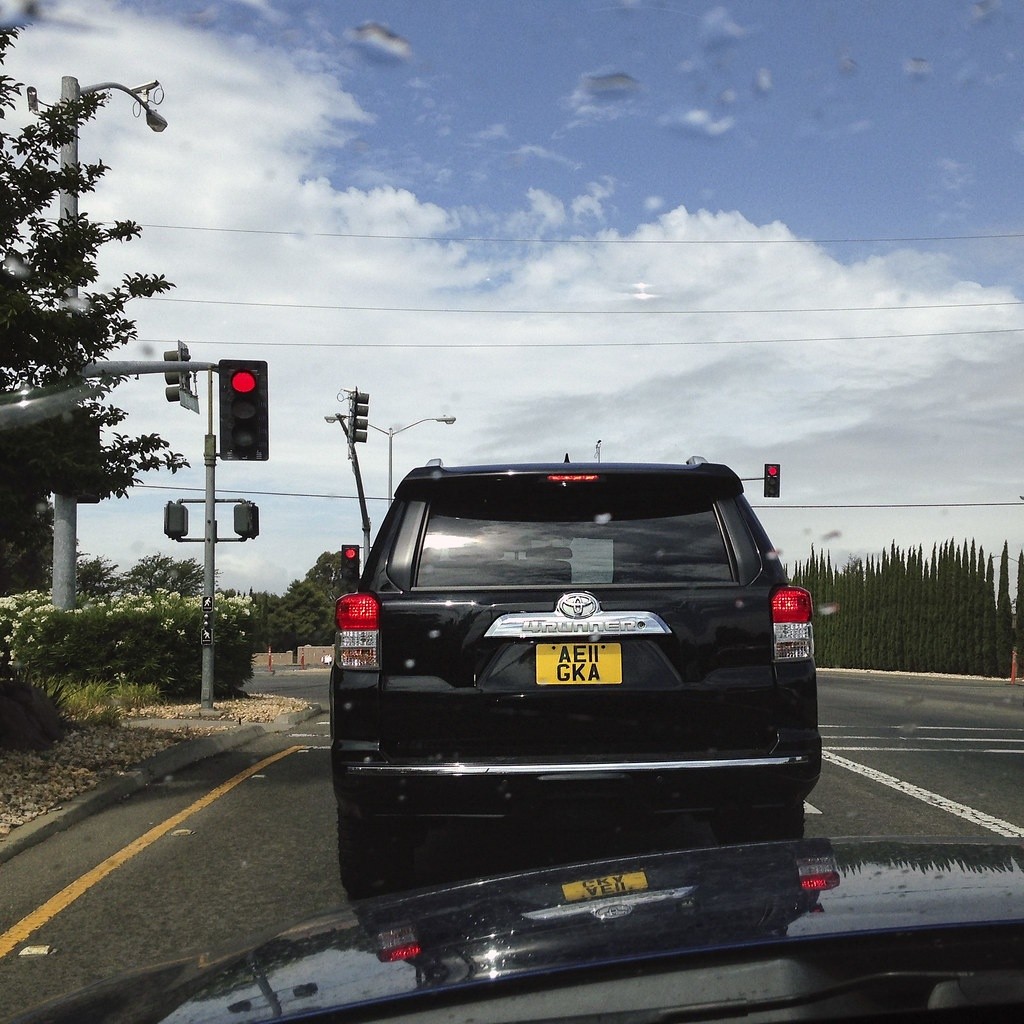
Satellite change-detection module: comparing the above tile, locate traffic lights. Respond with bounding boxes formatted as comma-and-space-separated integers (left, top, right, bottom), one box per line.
339, 541, 360, 595
163, 341, 191, 404
218, 358, 269, 461
345, 386, 371, 446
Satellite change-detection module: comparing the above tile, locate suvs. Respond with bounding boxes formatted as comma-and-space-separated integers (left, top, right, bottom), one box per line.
328, 453, 822, 893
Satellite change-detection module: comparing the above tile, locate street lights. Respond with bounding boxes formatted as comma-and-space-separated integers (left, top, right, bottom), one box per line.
764, 463, 781, 500
325, 415, 456, 509
